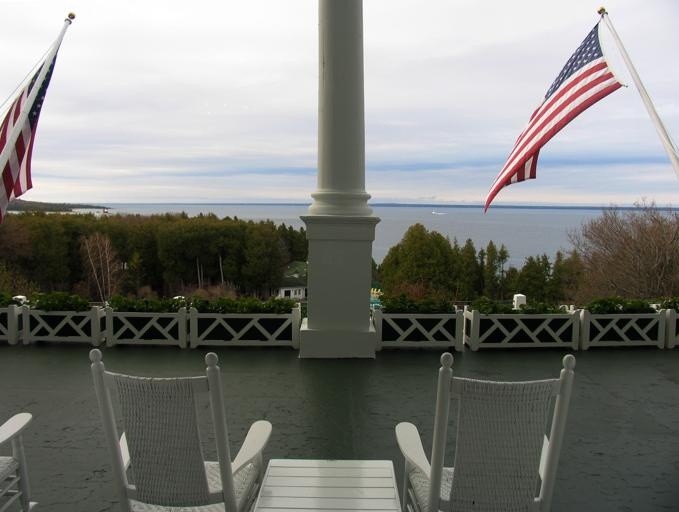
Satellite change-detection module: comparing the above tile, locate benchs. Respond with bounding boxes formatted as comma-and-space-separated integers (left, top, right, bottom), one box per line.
483, 5, 627, 213
0, 41, 58, 231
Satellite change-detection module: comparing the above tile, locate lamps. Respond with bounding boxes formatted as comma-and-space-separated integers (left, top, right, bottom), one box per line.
87, 348, 275, 512
397, 351, 576, 512
0, 413, 35, 511
